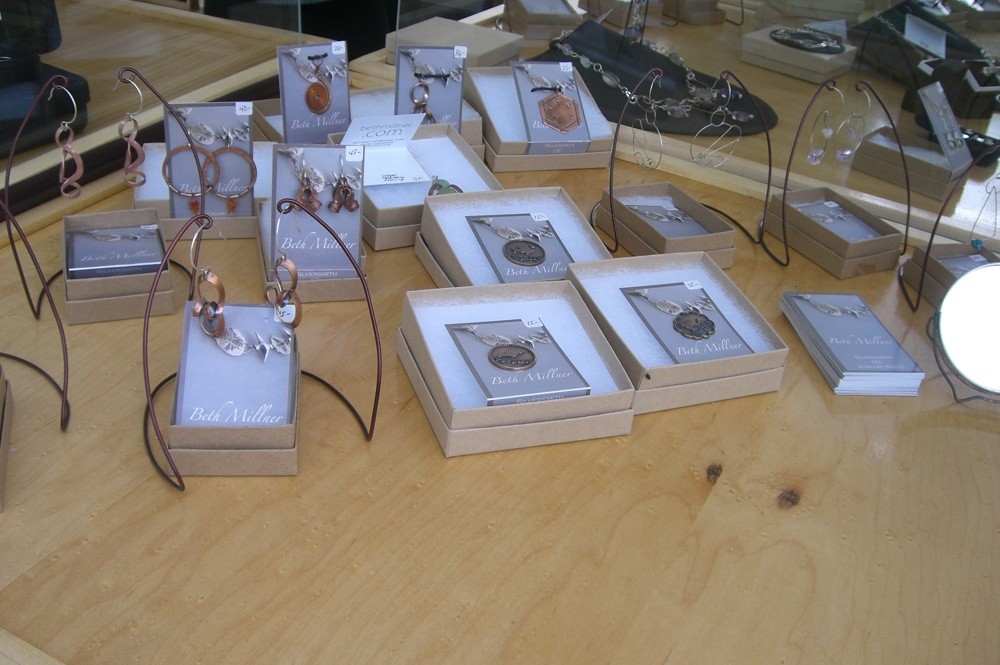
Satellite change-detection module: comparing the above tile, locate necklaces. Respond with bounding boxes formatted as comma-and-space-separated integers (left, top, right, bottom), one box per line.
517, 62, 583, 135
873, 10, 996, 65
452, 324, 551, 372
627, 287, 717, 340
961, 127, 994, 146
769, 27, 843, 49
174, 104, 249, 149
551, 29, 744, 119
277, 147, 364, 195
628, 204, 697, 224
791, 294, 873, 321
78, 229, 160, 243
283, 48, 348, 113
215, 327, 291, 362
400, 50, 462, 127
472, 217, 554, 266
808, 210, 853, 223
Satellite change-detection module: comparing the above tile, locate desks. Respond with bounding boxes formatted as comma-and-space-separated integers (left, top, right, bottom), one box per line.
0, 69, 1000, 665
348, 0, 1000, 249
0, 0, 340, 221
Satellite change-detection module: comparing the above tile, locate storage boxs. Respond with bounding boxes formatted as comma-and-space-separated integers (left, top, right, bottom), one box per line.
63, 0, 1000, 476
